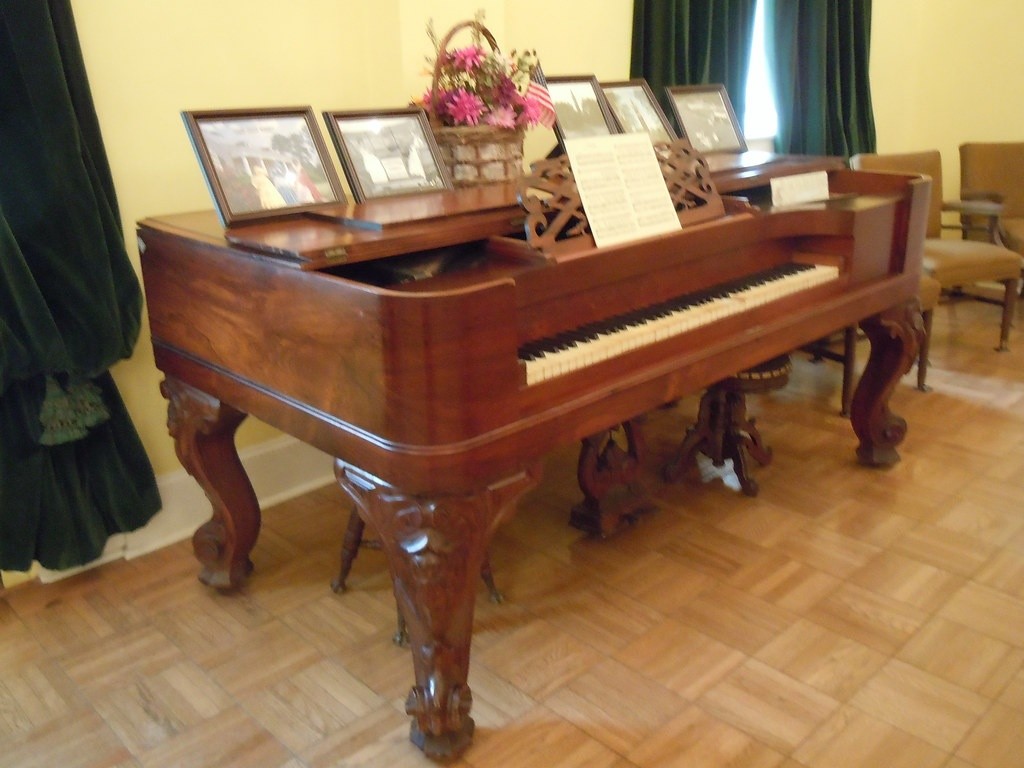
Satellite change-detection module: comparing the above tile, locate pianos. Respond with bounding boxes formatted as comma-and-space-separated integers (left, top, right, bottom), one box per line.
131, 148, 931, 763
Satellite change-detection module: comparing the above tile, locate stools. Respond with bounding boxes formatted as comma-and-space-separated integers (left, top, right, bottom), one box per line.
663, 353, 793, 497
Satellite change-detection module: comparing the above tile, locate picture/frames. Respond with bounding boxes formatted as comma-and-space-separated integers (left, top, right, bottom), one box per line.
664, 84, 748, 154
181, 106, 348, 230
599, 78, 686, 159
322, 107, 455, 205
541, 74, 619, 179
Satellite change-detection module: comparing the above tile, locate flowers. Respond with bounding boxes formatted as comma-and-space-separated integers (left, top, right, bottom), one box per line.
411, 9, 542, 131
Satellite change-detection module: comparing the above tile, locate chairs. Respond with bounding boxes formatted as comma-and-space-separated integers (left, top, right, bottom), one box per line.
795, 141, 1024, 418
